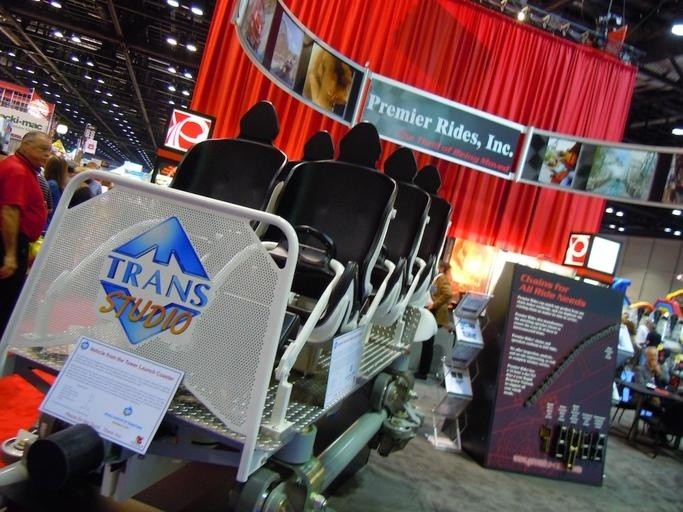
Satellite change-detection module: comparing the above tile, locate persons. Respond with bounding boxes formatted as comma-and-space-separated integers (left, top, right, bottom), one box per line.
612, 312, 683, 408
413, 261, 453, 381
0, 129, 114, 336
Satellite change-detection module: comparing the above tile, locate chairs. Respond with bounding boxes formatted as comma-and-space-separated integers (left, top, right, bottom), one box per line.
626, 386, 683, 457
612, 398, 636, 434
33, 101, 290, 355
394, 164, 453, 345
365, 147, 432, 348
264, 131, 334, 211
173, 119, 398, 442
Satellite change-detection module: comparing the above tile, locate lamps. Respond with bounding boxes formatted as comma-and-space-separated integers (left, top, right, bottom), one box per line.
519, 6, 528, 21
581, 30, 589, 44
500, 0, 507, 11
542, 15, 550, 29
560, 22, 570, 36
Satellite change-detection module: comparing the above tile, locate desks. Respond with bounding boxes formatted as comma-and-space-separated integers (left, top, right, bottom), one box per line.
611, 380, 683, 449
